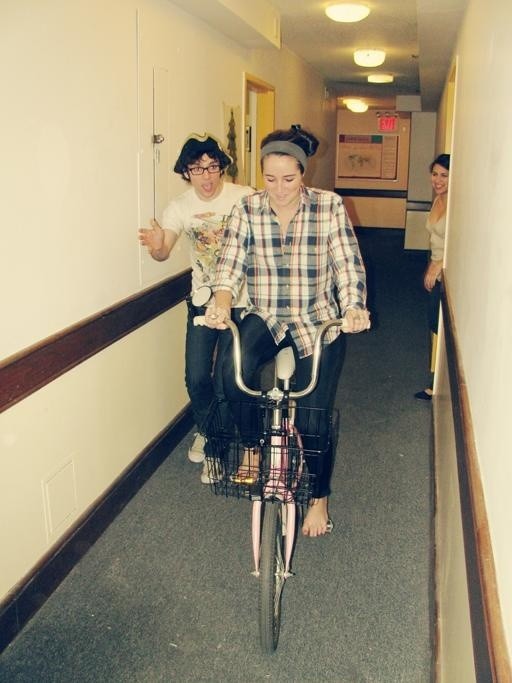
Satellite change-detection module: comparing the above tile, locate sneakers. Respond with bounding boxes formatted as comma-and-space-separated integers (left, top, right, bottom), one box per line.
187, 430, 226, 485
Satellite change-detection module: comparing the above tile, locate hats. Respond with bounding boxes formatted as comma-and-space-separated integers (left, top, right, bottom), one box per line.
173, 131, 234, 174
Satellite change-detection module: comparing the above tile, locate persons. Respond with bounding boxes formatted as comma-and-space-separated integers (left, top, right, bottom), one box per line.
204, 130, 374, 537
137, 130, 255, 483
413, 154, 451, 400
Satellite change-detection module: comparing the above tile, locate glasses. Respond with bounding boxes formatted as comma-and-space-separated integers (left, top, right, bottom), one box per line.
186, 164, 221, 175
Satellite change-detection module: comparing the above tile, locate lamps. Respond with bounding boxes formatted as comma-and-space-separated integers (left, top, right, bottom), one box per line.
325, 2, 394, 115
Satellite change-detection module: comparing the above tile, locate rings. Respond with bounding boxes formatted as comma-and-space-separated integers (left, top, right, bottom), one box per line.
353, 312, 360, 320
209, 313, 219, 320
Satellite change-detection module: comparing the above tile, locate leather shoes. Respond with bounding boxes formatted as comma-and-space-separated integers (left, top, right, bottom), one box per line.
414, 388, 432, 400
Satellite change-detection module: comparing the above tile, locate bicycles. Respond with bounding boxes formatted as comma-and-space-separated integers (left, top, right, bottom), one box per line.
191, 312, 373, 656
